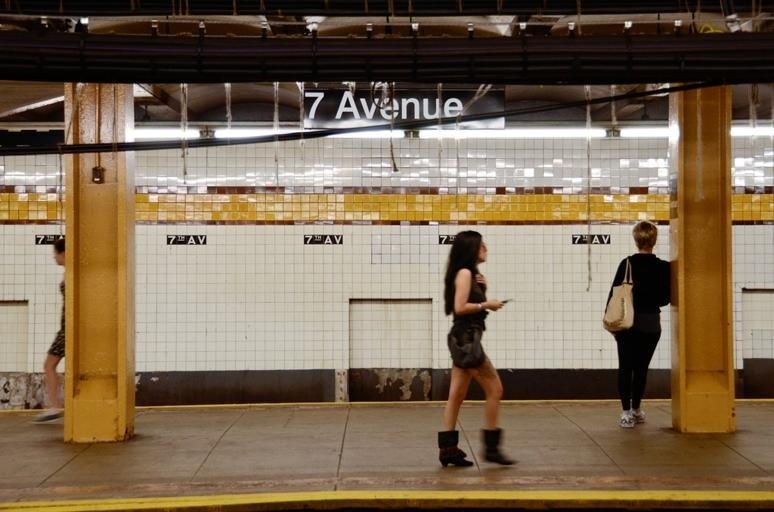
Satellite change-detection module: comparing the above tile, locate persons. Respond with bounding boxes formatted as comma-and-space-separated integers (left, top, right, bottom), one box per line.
435, 230, 521, 467
602, 221, 672, 429
29, 235, 64, 426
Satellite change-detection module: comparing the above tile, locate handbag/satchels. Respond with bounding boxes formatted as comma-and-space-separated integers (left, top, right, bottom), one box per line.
601, 281, 635, 333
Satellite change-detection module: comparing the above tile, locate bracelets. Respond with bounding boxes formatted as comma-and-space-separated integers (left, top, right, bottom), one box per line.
478, 303, 483, 312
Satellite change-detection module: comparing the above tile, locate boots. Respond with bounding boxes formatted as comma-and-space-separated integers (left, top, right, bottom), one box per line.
436, 429, 474, 468
479, 425, 521, 466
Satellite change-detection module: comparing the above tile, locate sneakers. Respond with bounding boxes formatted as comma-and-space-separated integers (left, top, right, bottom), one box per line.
632, 410, 648, 424
28, 409, 64, 428
619, 414, 635, 430
